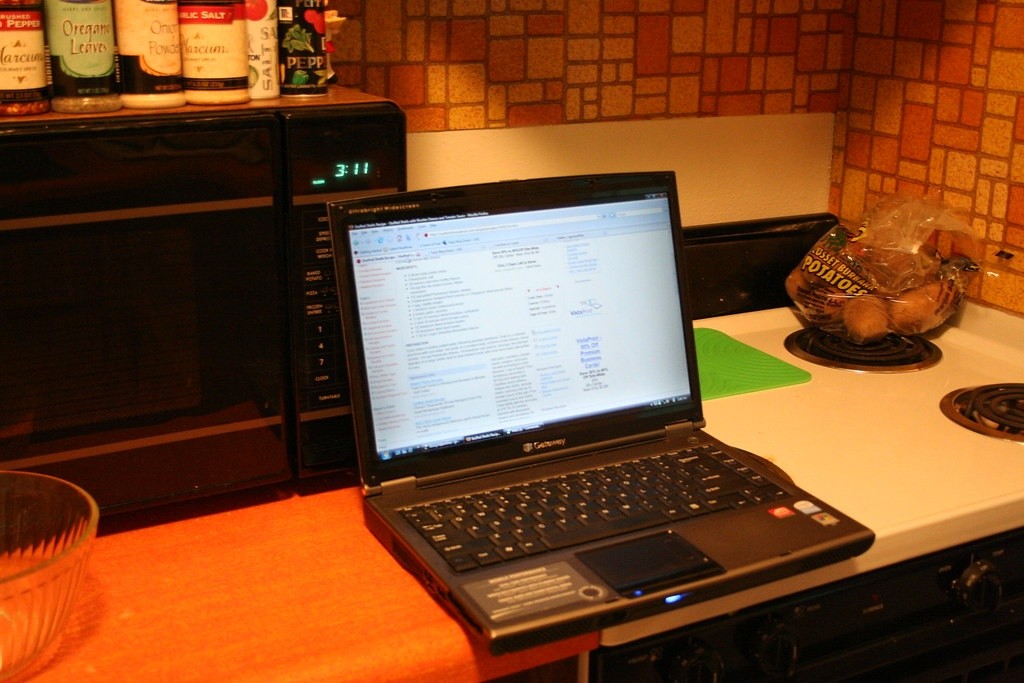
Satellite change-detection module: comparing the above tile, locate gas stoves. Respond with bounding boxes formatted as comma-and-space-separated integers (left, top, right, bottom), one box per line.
599, 210, 1024, 649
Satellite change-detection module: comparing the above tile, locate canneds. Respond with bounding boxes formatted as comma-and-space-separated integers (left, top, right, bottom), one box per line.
0, 0, 328, 116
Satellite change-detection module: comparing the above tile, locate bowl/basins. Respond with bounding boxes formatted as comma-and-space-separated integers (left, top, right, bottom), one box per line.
0, 468, 100, 680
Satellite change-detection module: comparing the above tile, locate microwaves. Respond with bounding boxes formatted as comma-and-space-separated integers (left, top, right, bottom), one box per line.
0, 85, 408, 538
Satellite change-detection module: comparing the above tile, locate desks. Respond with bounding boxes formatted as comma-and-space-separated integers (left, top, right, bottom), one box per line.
0, 484, 600, 683
598, 304, 1024, 646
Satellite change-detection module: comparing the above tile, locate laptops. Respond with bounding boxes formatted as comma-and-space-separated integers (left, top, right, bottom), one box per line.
326, 170, 877, 657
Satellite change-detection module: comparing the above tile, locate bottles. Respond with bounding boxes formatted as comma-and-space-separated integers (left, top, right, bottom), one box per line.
0, 0, 331, 116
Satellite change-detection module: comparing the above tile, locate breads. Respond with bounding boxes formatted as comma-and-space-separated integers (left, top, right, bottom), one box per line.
784, 222, 961, 342
